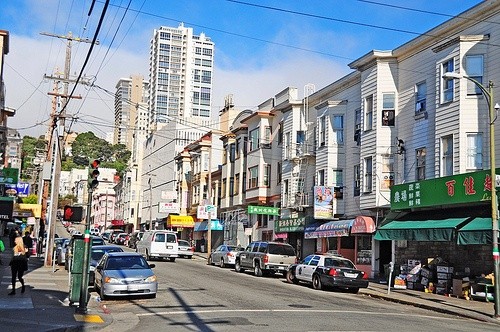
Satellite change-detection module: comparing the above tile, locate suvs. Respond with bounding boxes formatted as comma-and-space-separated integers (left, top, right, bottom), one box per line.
234, 241, 300, 277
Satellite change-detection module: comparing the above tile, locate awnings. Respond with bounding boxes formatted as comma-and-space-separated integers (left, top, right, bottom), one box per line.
305, 213, 500, 246
167, 215, 223, 231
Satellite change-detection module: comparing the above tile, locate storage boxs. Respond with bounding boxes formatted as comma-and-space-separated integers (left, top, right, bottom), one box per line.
393, 259, 493, 300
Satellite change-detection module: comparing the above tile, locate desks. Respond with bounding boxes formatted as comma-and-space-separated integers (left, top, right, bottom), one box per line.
477, 282, 494, 303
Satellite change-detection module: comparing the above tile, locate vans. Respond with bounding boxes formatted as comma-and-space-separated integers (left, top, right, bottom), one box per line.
136, 229, 178, 262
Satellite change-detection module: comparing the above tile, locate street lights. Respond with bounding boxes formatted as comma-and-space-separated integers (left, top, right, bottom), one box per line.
441, 72, 500, 318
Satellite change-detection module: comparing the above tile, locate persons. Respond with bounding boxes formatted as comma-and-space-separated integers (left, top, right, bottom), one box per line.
0, 226, 32, 295
201, 236, 205, 253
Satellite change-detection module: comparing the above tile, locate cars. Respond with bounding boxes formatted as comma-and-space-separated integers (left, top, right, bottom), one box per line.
178, 240, 195, 259
89, 245, 124, 285
208, 245, 245, 268
42, 210, 144, 272
286, 253, 369, 294
93, 251, 158, 300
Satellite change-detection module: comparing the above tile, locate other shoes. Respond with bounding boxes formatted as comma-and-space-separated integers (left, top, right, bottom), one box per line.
21, 285, 25, 293
8, 292, 16, 295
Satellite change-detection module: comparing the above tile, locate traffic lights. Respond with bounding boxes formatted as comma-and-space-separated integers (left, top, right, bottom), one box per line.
87, 158, 101, 189
62, 205, 83, 221
